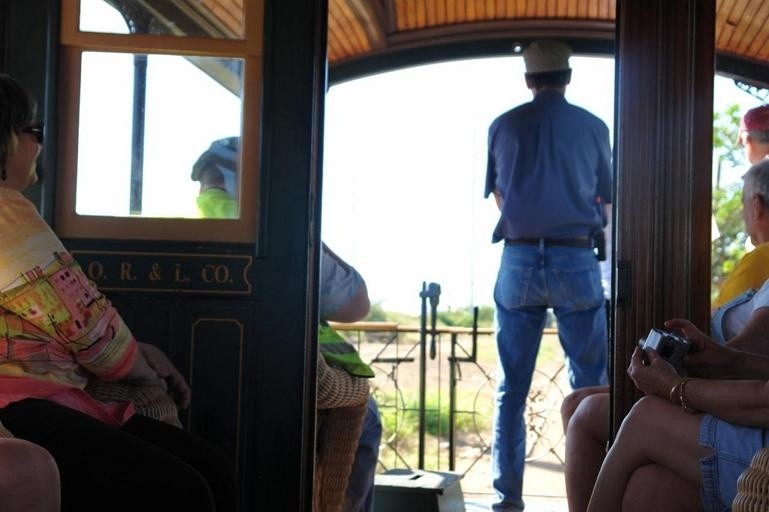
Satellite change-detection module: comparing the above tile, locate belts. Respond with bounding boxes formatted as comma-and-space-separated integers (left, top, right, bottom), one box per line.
503, 235, 598, 248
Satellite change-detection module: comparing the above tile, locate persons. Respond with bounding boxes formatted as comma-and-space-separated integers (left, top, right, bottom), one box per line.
188, 134, 382, 512
729, 104, 769, 171
709, 159, 768, 315
478, 38, 621, 512
559, 277, 769, 512
585, 315, 768, 511
2, 82, 222, 512
1, 424, 63, 512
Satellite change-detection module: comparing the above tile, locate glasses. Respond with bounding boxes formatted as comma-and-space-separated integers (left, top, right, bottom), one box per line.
13, 120, 49, 144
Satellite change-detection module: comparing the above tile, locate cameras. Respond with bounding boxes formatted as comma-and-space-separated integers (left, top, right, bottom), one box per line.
638, 328, 691, 368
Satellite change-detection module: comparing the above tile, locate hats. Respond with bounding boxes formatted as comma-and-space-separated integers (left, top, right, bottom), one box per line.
732, 104, 769, 149
524, 40, 574, 75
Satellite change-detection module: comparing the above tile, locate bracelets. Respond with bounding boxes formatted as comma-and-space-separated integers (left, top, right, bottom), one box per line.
667, 379, 681, 402
680, 376, 697, 415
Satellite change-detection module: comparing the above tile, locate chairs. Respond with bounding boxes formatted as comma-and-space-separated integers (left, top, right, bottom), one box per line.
85, 377, 181, 429
313, 357, 367, 510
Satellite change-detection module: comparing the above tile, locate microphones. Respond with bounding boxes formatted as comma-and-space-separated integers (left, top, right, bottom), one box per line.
430, 282, 441, 361
